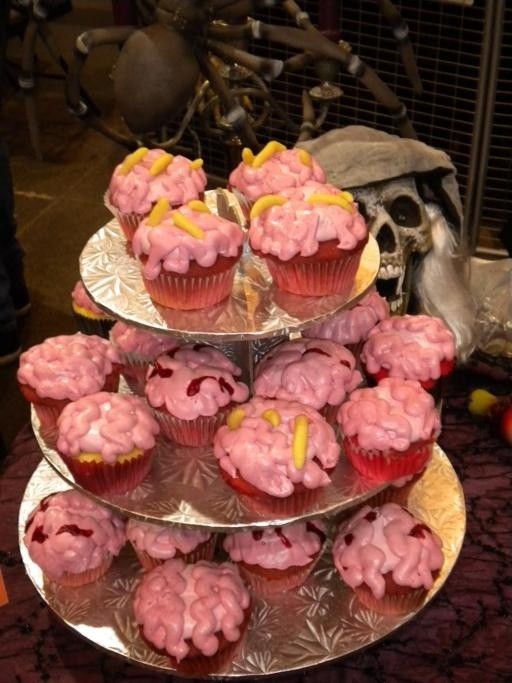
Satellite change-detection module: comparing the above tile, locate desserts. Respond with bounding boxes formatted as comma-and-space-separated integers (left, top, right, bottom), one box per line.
24, 465, 445, 664
15, 280, 459, 505
105, 139, 371, 309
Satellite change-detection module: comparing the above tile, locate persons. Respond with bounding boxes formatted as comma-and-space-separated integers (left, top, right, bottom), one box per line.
0, 148, 31, 368
